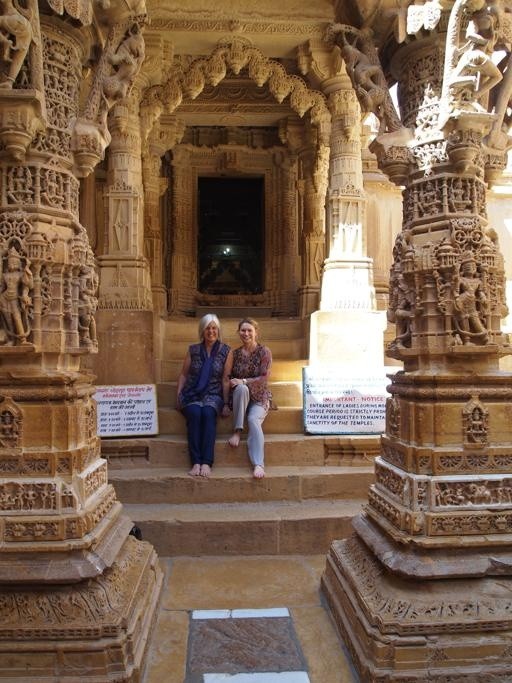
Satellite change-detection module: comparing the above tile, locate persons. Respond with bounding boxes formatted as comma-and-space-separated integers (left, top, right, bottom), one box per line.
174, 311, 235, 477
226, 316, 279, 478
457, 251, 493, 344
0, 246, 38, 345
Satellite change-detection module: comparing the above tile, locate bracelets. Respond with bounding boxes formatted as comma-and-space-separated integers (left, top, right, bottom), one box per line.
242, 377, 246, 384
